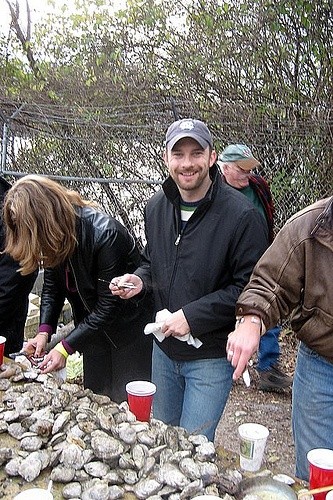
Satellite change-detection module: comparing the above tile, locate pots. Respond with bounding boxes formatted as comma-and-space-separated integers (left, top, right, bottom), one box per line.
233, 476, 333, 500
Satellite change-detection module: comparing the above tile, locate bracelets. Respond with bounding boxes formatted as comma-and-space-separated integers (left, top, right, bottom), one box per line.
241, 316, 261, 323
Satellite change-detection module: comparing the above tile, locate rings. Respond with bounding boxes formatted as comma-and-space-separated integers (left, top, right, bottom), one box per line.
228, 350, 234, 356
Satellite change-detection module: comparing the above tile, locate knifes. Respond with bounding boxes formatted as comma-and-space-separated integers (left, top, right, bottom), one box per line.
228, 332, 252, 388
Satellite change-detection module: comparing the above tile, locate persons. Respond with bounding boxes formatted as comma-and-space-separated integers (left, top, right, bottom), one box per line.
226, 194, 333, 483
0, 174, 152, 408
218, 143, 293, 391
110, 119, 268, 442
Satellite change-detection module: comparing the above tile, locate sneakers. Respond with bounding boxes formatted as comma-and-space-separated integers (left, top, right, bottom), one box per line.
261, 368, 294, 389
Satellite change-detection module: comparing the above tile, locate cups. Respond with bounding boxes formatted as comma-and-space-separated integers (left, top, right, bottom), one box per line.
307, 448, 333, 500
0, 336, 7, 366
237, 423, 270, 471
126, 380, 157, 422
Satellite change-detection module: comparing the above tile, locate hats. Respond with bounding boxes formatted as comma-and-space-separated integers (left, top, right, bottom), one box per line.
166, 119, 213, 152
220, 144, 262, 171
